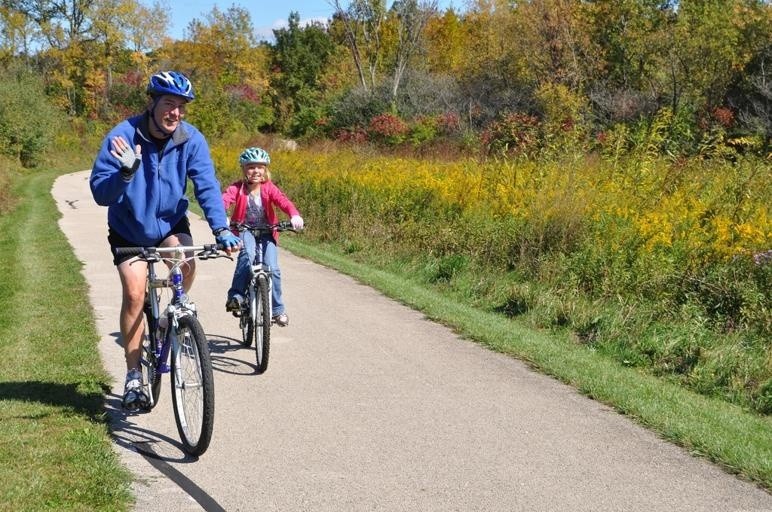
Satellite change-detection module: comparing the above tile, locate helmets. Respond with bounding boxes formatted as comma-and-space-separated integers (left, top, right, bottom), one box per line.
239, 147, 270, 167
147, 71, 195, 103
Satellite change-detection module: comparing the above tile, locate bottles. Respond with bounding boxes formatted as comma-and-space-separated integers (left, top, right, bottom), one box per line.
156, 308, 169, 341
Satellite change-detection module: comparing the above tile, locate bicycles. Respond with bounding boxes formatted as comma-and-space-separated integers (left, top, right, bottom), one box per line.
110, 237, 240, 460
225, 218, 310, 376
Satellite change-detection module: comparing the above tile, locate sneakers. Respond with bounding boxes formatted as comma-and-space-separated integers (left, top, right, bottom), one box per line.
274, 311, 289, 327
123, 368, 147, 408
225, 297, 242, 312
